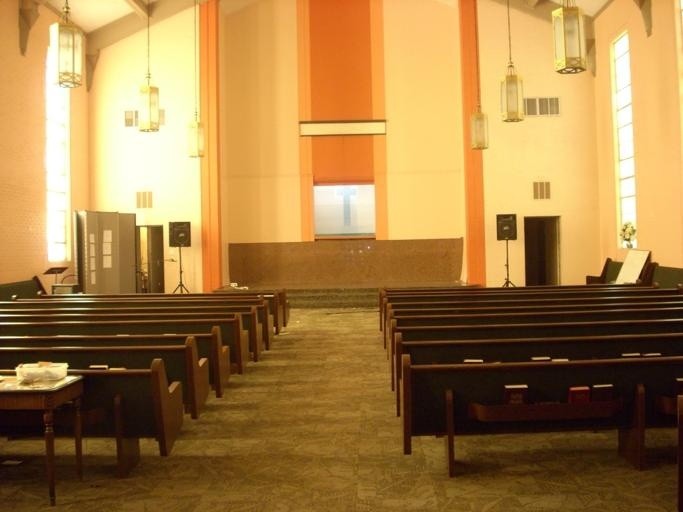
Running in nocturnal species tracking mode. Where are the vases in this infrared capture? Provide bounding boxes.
[626,242,633,248]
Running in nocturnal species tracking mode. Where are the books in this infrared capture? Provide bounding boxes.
[641,352,662,358]
[463,358,483,364]
[592,383,614,400]
[620,352,640,359]
[551,358,570,363]
[88,364,108,371]
[109,367,127,370]
[566,385,590,404]
[673,377,682,398]
[530,356,551,362]
[502,383,528,405]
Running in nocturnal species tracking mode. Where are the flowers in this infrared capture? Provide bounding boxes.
[619,222,637,242]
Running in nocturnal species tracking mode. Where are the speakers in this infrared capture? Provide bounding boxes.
[496,214,517,240]
[169,222,190,247]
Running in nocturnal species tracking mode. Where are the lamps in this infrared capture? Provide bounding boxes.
[49,1,207,159]
[468,0,587,149]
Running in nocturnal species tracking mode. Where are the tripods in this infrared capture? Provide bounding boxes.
[173,247,190,294]
[502,241,516,287]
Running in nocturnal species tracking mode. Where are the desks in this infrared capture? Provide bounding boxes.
[0,374,85,506]
